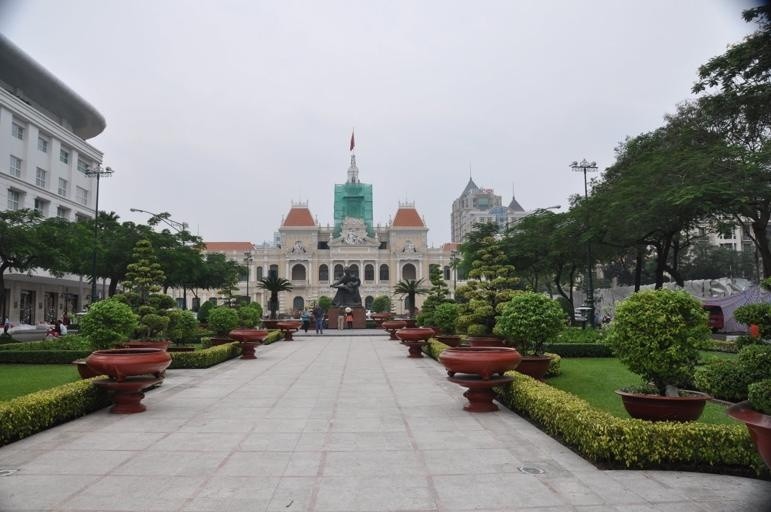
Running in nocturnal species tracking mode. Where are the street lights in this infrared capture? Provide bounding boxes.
[448,248,459,290]
[129,206,188,310]
[569,158,598,327]
[84,161,116,300]
[242,249,254,305]
[58,288,72,313]
[506,204,563,236]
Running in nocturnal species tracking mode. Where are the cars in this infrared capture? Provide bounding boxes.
[263,312,290,320]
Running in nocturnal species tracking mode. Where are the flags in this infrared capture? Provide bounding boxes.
[350,131,354,151]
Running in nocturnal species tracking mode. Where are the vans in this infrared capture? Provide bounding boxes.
[701,304,724,331]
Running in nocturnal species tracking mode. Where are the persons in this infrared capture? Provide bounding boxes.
[347,228,358,244]
[52,318,61,335]
[330,267,362,308]
[58,320,67,336]
[337,305,345,330]
[62,311,68,325]
[312,304,324,334]
[345,307,354,328]
[4,316,12,338]
[301,306,311,332]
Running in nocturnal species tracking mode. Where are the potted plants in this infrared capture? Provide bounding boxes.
[71,232,263,380]
[727,303,771,470]
[415,239,571,383]
[599,287,712,423]
